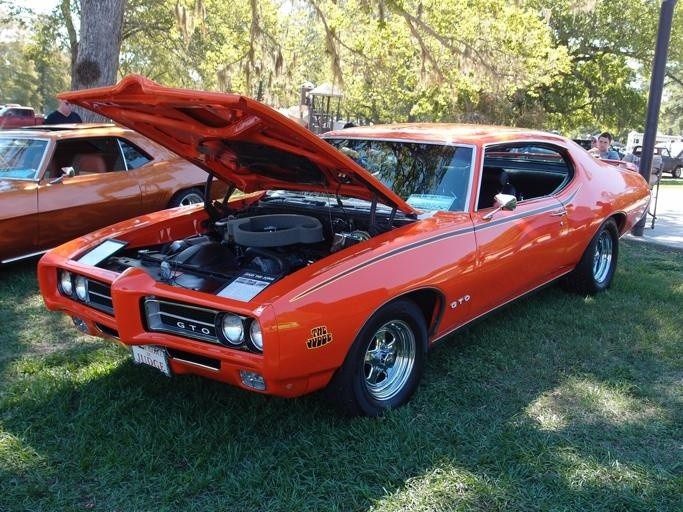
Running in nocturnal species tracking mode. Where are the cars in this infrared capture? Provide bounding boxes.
[576,129,682,179]
[33,73,653,415]
[0,123,230,271]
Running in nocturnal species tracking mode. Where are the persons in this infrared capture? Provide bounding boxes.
[585,132,620,162]
[43,98,82,122]
[590,134,600,149]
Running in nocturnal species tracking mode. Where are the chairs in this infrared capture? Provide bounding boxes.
[70,151,107,176]
[32,149,56,177]
[478,167,517,209]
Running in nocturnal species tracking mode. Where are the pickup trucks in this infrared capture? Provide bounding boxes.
[0,105,45,130]
